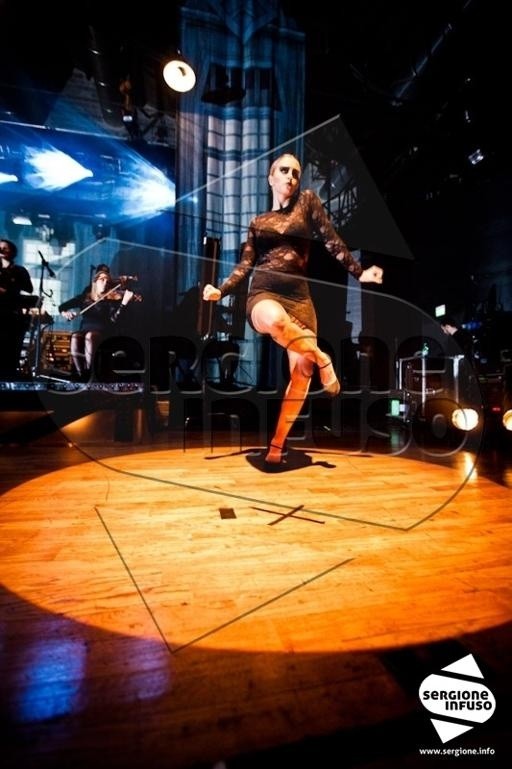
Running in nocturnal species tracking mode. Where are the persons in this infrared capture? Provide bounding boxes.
[176,285,240,387]
[439,317,482,405]
[201,150,387,474]
[82,264,129,298]
[57,270,134,383]
[1,237,33,381]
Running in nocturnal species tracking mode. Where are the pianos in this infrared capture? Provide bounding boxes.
[0,309,54,324]
[395,354,480,371]
[0,293,42,308]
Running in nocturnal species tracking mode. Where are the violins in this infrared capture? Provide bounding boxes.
[99,291,142,301]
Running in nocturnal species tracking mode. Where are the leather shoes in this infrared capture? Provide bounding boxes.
[317,352,342,400]
[263,441,283,472]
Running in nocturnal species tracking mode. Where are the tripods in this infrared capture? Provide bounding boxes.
[15,251,72,383]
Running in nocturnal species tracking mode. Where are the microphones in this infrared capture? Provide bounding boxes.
[45,265,56,279]
